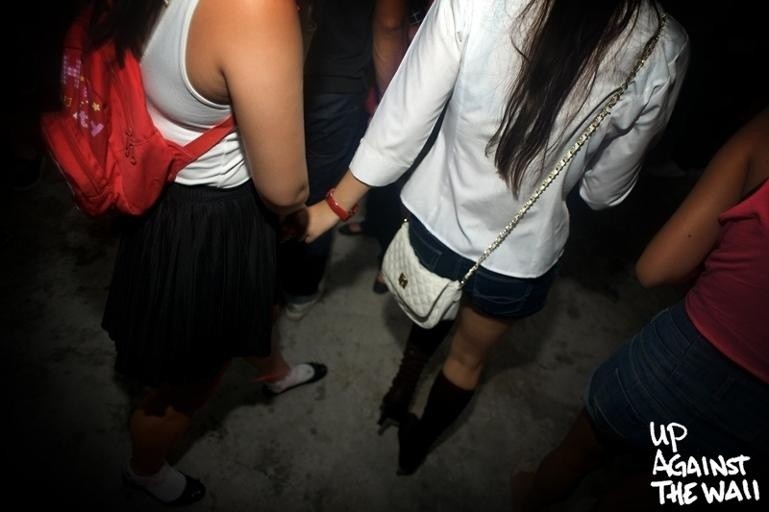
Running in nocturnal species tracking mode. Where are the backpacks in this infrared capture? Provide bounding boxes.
[38,0,238,219]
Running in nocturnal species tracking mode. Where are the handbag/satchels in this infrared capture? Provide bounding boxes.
[380,222,463,330]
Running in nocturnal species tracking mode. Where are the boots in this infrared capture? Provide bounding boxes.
[377,320,475,475]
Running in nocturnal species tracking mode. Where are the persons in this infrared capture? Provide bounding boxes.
[0,0,769,512]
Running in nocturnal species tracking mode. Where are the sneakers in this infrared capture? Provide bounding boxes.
[285,276,326,321]
[372,274,386,295]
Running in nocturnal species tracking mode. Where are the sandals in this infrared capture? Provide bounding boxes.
[338,222,370,235]
[119,471,204,506]
[262,363,328,396]
